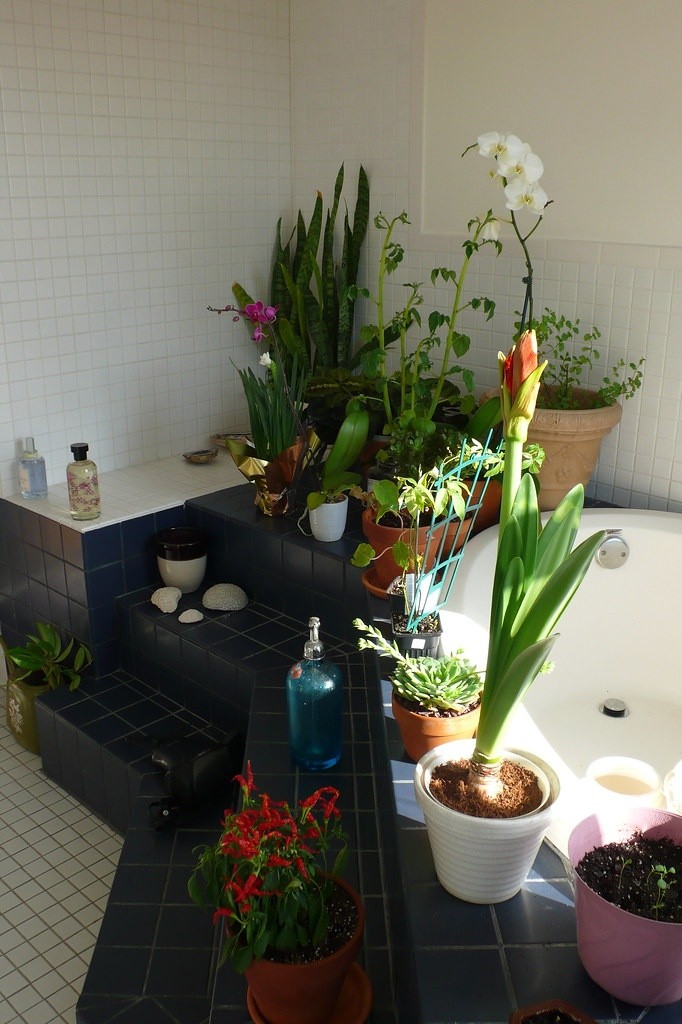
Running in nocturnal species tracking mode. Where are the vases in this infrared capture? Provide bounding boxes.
[305,493,356,542]
[414,739,562,904]
[156,526,210,596]
[479,385,624,511]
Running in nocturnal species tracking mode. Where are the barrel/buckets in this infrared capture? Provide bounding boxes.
[157,528,206,594]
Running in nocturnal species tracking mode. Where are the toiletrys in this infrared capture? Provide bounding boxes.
[16,431,49,503]
[285,614,347,774]
[61,439,103,523]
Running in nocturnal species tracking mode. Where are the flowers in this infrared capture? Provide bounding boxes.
[205,300,368,511]
[468,325,622,799]
[463,130,649,410]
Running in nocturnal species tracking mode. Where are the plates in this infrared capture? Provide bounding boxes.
[212,432,253,448]
[183,449,217,463]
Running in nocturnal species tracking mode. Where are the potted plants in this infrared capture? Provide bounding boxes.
[188,760,372,1024]
[568,805,682,1007]
[0,620,96,755]
[230,160,576,771]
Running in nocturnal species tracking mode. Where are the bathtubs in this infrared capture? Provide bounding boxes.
[434,507,681,855]
